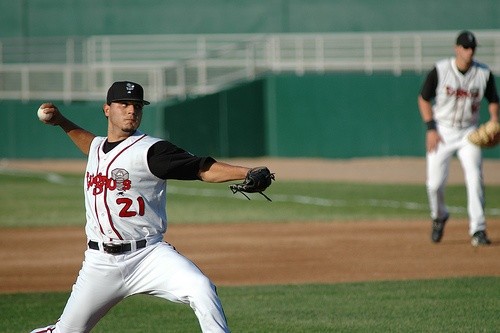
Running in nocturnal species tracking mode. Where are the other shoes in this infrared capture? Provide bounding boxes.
[431,213,449,242]
[471,232,491,246]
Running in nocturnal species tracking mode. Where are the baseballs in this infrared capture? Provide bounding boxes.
[37,105,53,122]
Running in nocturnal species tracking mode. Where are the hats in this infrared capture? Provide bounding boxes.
[107,82,150,105]
[457,31,476,47]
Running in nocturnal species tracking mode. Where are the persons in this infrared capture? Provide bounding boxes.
[417,32,500,245]
[30,81,272,333]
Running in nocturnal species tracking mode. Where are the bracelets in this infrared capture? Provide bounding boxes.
[426,120,436,130]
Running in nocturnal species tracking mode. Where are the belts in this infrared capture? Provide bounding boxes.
[88,240,146,255]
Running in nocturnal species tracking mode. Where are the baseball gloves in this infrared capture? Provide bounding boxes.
[468,120,500,149]
[228,168,272,194]
